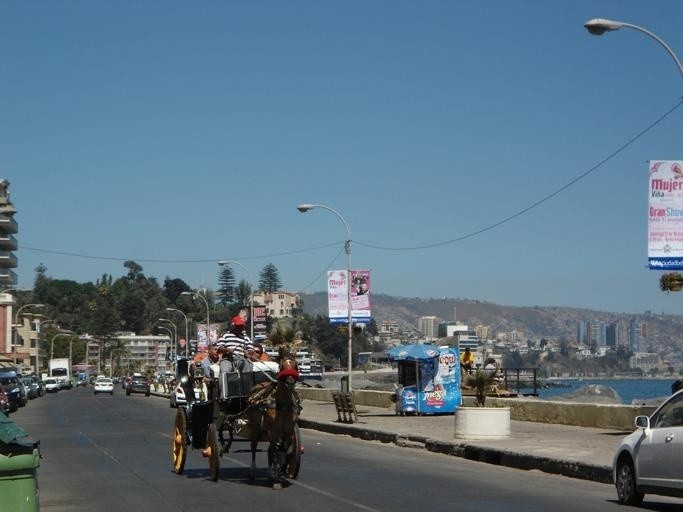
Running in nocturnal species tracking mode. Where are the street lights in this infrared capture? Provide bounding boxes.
[217,260,254,342]
[0,288,122,377]
[156,287,210,358]
[296,204,353,394]
[585,18,683,80]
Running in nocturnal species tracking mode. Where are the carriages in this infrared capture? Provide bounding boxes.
[171,347,304,489]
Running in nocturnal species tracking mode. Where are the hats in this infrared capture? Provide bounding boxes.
[277,357,300,381]
[230,316,245,326]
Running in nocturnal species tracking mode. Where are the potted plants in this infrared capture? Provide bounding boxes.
[452,368,512,441]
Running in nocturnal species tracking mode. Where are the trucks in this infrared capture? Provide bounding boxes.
[48,357,72,389]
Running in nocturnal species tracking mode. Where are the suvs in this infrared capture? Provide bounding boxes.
[125,376,150,397]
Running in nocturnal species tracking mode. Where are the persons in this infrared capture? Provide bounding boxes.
[200,342,220,384]
[250,343,262,362]
[459,346,474,370]
[483,357,504,385]
[215,315,255,406]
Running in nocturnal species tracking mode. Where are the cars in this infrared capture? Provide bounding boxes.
[0,367,44,415]
[43,377,59,392]
[76,369,141,388]
[611,387,683,506]
[169,380,202,408]
[93,378,113,395]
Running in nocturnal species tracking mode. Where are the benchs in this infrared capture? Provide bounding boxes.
[332,393,370,425]
[207,361,280,397]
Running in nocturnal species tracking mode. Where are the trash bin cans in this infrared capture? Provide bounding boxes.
[0,449,39,512]
